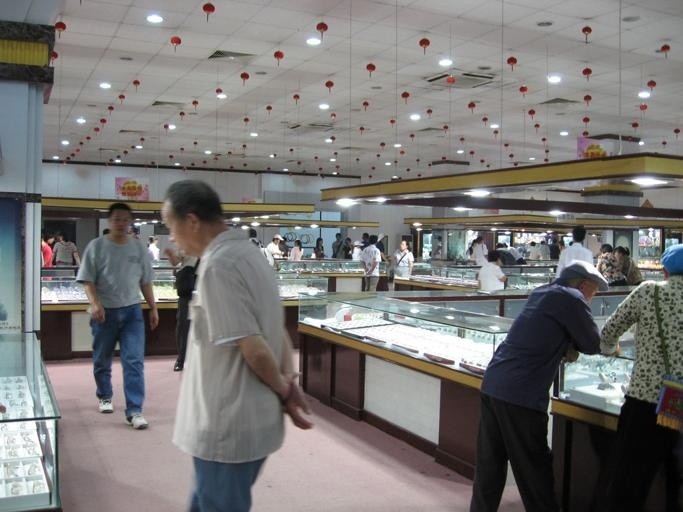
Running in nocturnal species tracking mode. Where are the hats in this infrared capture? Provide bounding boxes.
[377,234,385,242]
[274,234,284,241]
[560,261,609,291]
[353,241,365,246]
[660,244,683,273]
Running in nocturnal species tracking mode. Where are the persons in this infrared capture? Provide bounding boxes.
[166,246,199,373]
[392,241,414,275]
[75,204,160,430]
[468,256,610,511]
[147,236,159,261]
[598,245,644,287]
[160,179,314,511]
[290,240,304,260]
[314,238,327,259]
[477,251,507,289]
[495,239,573,265]
[249,229,288,266]
[466,236,488,264]
[41,229,80,281]
[555,225,594,280]
[332,232,387,291]
[127,228,140,240]
[103,229,110,235]
[597,244,682,512]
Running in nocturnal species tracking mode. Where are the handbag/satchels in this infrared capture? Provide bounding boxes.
[176,266,198,299]
[657,375,683,432]
[389,270,394,282]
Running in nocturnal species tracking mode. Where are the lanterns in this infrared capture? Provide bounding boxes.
[662,128,680,148]
[65,3,216,168]
[630,42,669,144]
[216,21,550,179]
[50,20,67,66]
[581,24,592,137]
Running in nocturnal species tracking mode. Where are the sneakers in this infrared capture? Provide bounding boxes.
[98,398,114,413]
[128,413,147,429]
